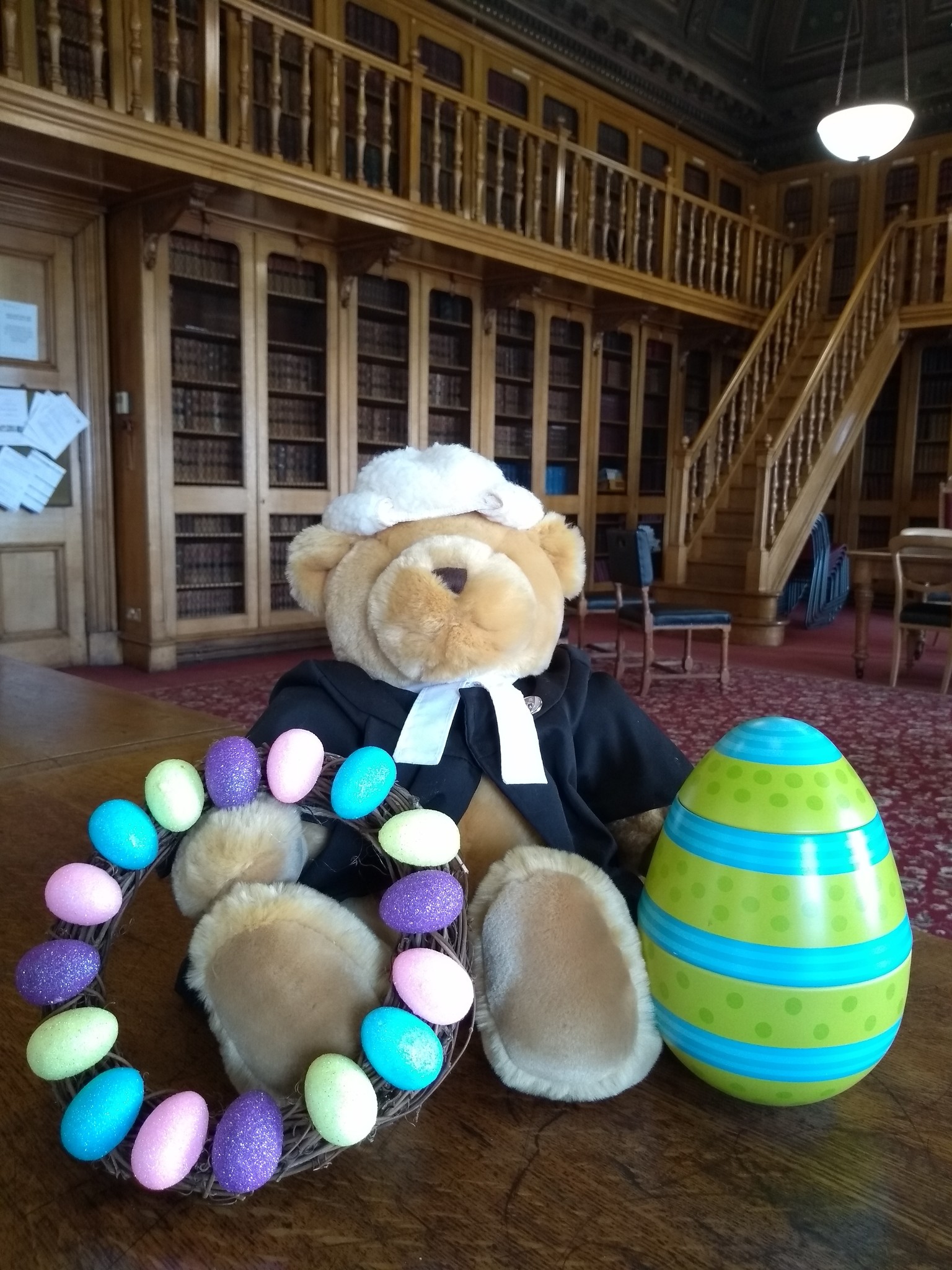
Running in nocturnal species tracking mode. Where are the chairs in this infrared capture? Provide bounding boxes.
[778,512,855,632]
[564,586,656,675]
[888,525,952,698]
[606,528,733,702]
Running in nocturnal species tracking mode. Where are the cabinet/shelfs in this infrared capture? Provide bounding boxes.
[0,0,767,661]
[776,135,952,605]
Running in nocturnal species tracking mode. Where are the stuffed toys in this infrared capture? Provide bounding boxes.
[171,439,699,1109]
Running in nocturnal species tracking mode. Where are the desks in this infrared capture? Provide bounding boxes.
[846,544,951,680]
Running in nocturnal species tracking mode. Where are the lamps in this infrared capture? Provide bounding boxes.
[817,0,915,162]
